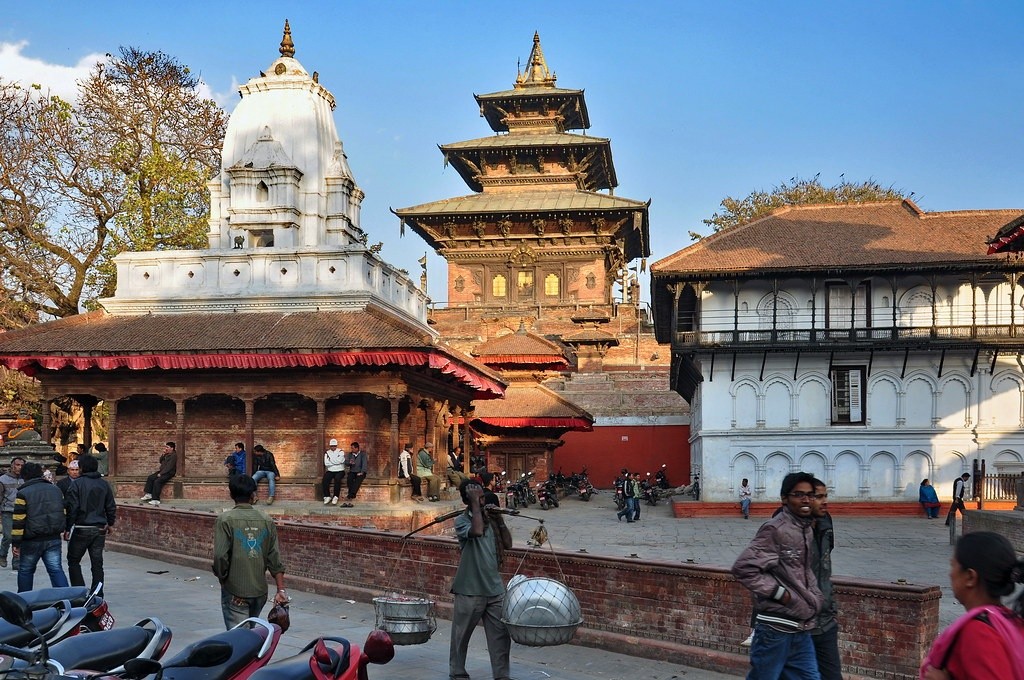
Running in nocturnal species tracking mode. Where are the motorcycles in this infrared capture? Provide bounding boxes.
[612,462,668,510]
[506,464,593,510]
[0,582,394,680]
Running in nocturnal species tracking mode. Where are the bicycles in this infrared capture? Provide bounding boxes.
[689,471,701,500]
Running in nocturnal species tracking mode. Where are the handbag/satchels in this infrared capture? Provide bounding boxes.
[268,601,290,634]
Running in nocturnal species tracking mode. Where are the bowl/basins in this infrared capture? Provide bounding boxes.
[373,597,436,620]
[379,620,434,646]
[502,576,581,625]
[501,617,585,647]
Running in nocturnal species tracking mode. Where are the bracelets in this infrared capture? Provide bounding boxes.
[277,585,285,593]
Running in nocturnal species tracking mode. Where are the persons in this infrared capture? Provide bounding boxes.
[740,478,843,680]
[344,442,368,501]
[617,472,641,523]
[731,471,825,680]
[945,473,970,525]
[0,442,116,600]
[322,439,346,505]
[481,472,501,508]
[739,478,752,519]
[397,443,424,502]
[141,442,177,505]
[210,474,291,630]
[921,530,1024,680]
[250,444,280,505]
[447,447,468,488]
[919,479,941,519]
[448,480,513,680]
[416,442,441,502]
[223,442,246,475]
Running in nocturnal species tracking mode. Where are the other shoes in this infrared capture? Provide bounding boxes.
[266,497,274,505]
[617,512,622,521]
[12,562,20,570]
[141,493,152,500]
[928,516,932,519]
[410,494,424,503]
[633,518,640,520]
[0,556,7,567]
[340,502,354,508]
[148,500,160,505]
[332,496,339,505]
[324,497,332,505]
[627,520,636,523]
[426,495,438,502]
[253,496,259,504]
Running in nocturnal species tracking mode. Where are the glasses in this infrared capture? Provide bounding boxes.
[787,490,816,500]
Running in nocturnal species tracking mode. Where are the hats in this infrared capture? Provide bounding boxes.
[329,439,337,446]
[405,442,413,448]
[68,460,79,468]
[424,442,434,448]
